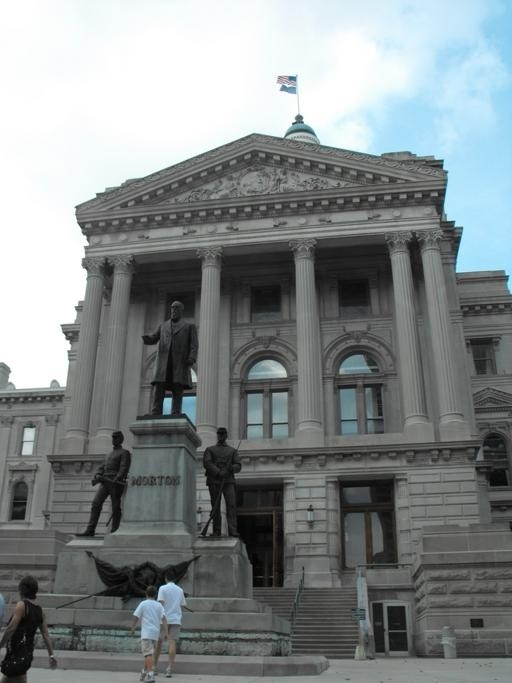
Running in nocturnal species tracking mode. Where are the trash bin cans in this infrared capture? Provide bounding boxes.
[442,626,457,659]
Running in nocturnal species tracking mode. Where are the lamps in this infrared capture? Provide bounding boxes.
[196,506,203,527]
[305,504,314,528]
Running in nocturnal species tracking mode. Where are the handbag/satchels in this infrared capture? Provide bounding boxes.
[0,637,34,677]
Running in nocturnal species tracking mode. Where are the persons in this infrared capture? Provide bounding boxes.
[202,427,243,538]
[141,300,199,416]
[130,570,188,683]
[75,430,132,538]
[0,575,58,683]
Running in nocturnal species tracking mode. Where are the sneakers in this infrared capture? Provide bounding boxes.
[166,669,172,676]
[139,667,157,682]
